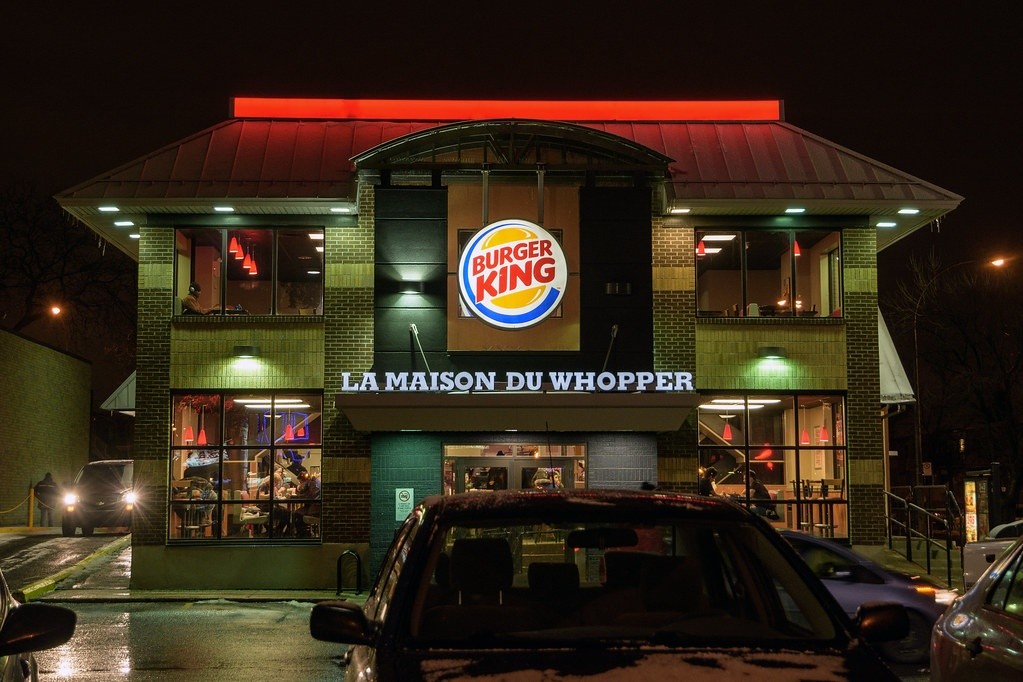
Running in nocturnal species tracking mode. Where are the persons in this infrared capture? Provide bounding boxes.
[535,469,566,490]
[293,471,321,538]
[182,282,209,315]
[34,472,59,528]
[742,470,774,516]
[287,455,308,477]
[254,471,291,538]
[699,464,726,500]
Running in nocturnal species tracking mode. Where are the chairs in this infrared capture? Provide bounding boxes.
[302,515,321,538]
[789,479,846,538]
[232,490,269,537]
[298,308,316,315]
[422,538,545,637]
[769,490,787,529]
[597,548,704,620]
[724,303,740,317]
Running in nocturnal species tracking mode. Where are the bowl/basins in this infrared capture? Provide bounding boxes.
[730,494,739,497]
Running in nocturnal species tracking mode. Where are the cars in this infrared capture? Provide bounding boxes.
[779,529,1023,682]
[0,568,77,682]
[311,491,902,682]
[62,460,135,537]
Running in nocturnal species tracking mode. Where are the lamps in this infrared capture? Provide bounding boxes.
[186,402,194,442]
[801,405,811,445]
[756,346,787,359]
[697,240,706,256]
[284,408,295,441]
[723,410,732,440]
[606,282,631,295]
[794,240,801,256]
[198,404,208,445]
[820,401,828,443]
[233,345,261,357]
[229,229,257,275]
[297,427,305,437]
[397,280,425,294]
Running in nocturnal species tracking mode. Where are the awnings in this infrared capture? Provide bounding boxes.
[100,369,136,417]
[877,303,917,407]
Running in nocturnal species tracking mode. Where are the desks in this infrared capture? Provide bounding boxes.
[759,310,817,317]
[210,309,248,316]
[696,309,723,317]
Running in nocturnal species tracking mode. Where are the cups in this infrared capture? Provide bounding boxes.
[722,491,726,496]
[287,487,296,496]
